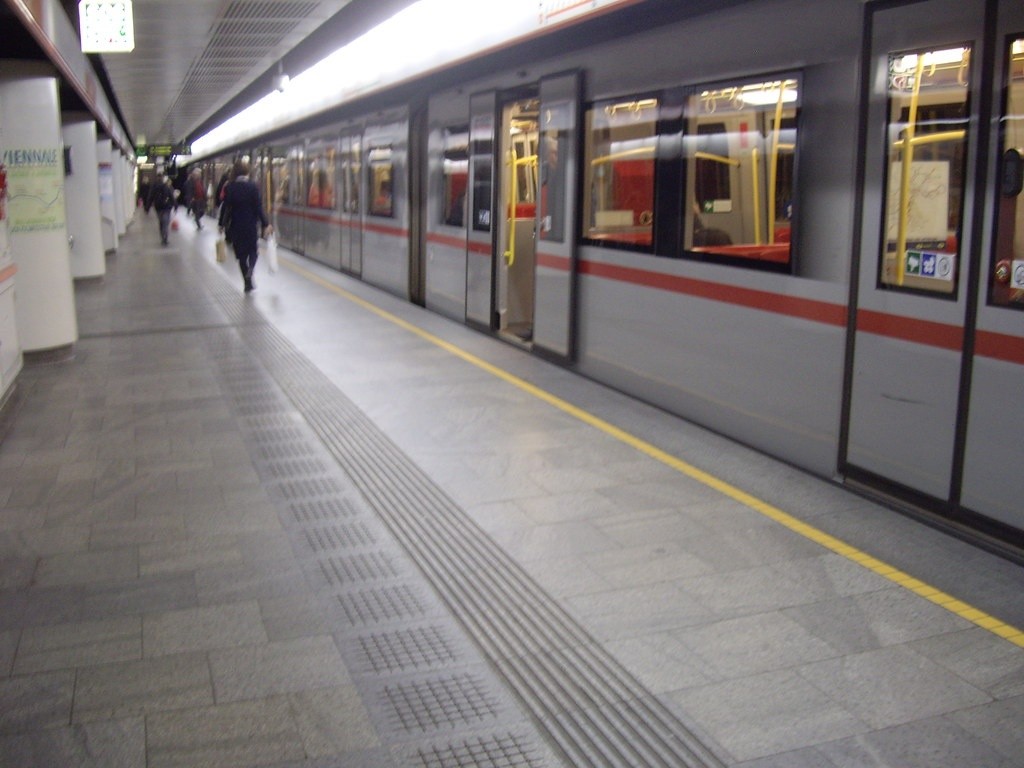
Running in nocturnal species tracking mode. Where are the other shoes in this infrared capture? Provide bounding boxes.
[244,278,253,291]
[162,236,168,246]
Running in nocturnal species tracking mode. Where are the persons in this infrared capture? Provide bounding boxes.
[218,163,273,293]
[215,167,238,260]
[148,173,178,247]
[189,168,209,230]
[276,165,332,255]
[140,175,153,213]
[183,175,193,216]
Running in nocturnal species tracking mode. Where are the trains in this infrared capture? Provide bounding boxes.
[176,2,1024,565]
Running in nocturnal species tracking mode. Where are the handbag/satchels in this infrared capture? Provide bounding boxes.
[171,213,180,230]
[267,231,278,272]
[216,232,226,261]
